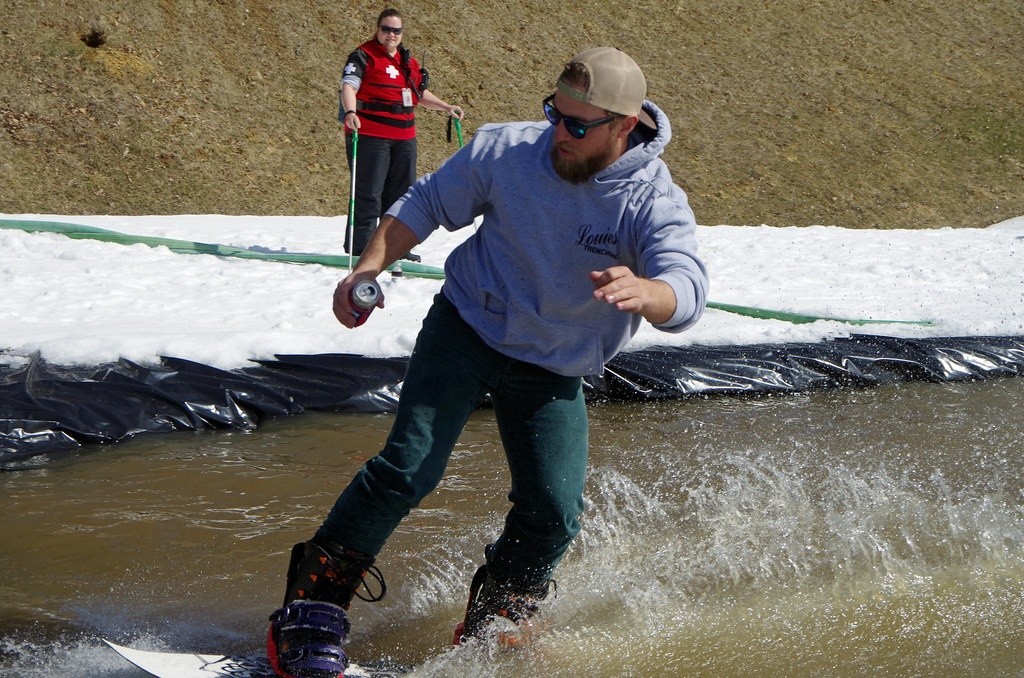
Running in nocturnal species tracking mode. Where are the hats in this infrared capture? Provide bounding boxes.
[557,47,657,130]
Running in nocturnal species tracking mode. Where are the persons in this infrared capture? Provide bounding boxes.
[340,9,464,263]
[268,48,708,678]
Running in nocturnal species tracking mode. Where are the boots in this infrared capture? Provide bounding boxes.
[272,542,386,678]
[464,544,559,651]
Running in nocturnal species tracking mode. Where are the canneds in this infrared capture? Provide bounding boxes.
[349,281,381,327]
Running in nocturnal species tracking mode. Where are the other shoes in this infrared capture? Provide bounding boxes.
[400,253,421,262]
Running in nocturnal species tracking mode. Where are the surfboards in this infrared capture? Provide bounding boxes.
[101,636,540,678]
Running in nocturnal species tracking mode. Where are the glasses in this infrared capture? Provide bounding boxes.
[378,25,404,35]
[543,92,615,139]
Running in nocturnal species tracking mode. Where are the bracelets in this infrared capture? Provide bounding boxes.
[344,110,357,118]
